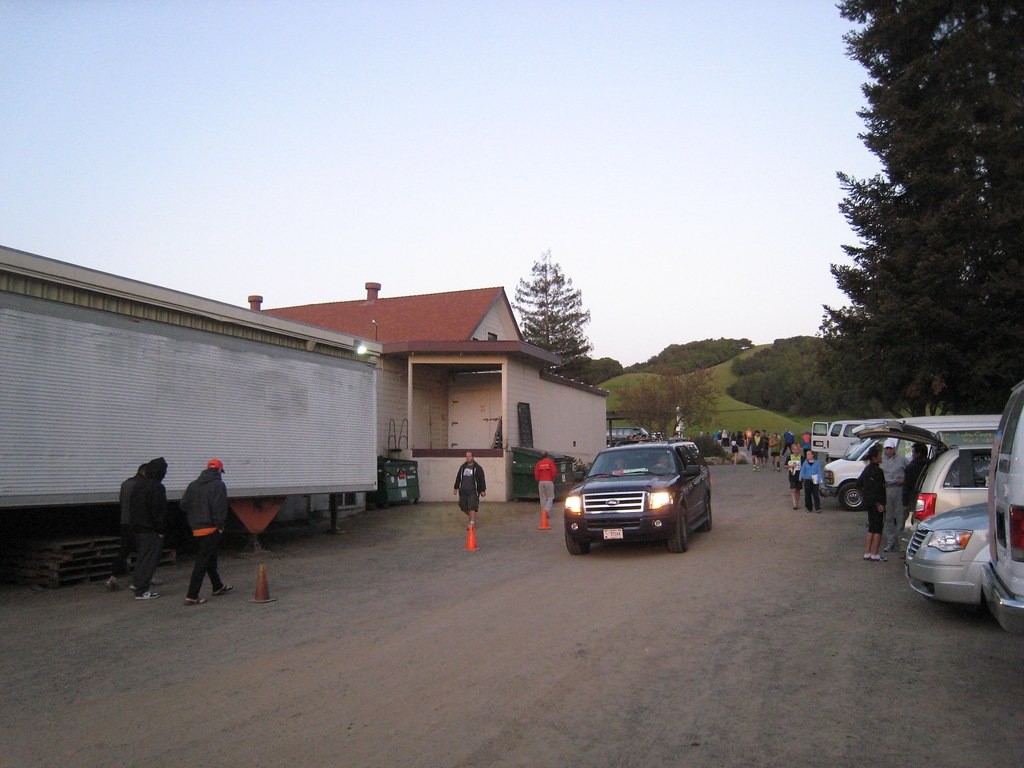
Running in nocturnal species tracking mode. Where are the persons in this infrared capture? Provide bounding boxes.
[130,457,168,600]
[180,459,234,606]
[732,443,738,466]
[714,427,753,450]
[454,451,486,531]
[802,430,811,457]
[656,455,669,470]
[902,443,928,512]
[799,449,822,513]
[747,429,781,473]
[780,429,794,458]
[613,455,628,470]
[674,433,678,438]
[785,444,803,510]
[879,439,910,553]
[627,435,646,440]
[688,436,692,442]
[104,463,163,591]
[857,447,888,562]
[535,453,557,519]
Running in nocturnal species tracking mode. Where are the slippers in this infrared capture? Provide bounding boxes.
[186,597,208,606]
[213,584,234,596]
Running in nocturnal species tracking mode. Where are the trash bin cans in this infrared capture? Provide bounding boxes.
[365,456,420,509]
[511,446,575,499]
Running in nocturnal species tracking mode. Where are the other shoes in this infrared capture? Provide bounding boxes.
[863,555,871,559]
[793,504,800,509]
[105,579,119,590]
[817,508,823,512]
[870,555,888,561]
[807,507,813,512]
[750,462,781,472]
[148,578,162,585]
[467,521,476,530]
[884,543,896,551]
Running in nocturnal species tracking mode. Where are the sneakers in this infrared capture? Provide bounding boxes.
[134,590,160,600]
[129,584,136,589]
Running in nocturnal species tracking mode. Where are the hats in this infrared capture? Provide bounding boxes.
[207,458,225,473]
[882,440,896,449]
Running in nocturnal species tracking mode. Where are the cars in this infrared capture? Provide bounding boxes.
[902,500,992,626]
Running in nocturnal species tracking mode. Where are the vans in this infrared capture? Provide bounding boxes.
[810,413,1007,541]
[979,379,1024,638]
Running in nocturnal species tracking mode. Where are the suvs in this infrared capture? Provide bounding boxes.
[562,436,714,555]
[605,427,648,447]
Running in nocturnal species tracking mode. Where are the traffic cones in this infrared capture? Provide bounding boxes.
[460,521,481,552]
[249,563,276,604]
[537,507,553,530]
